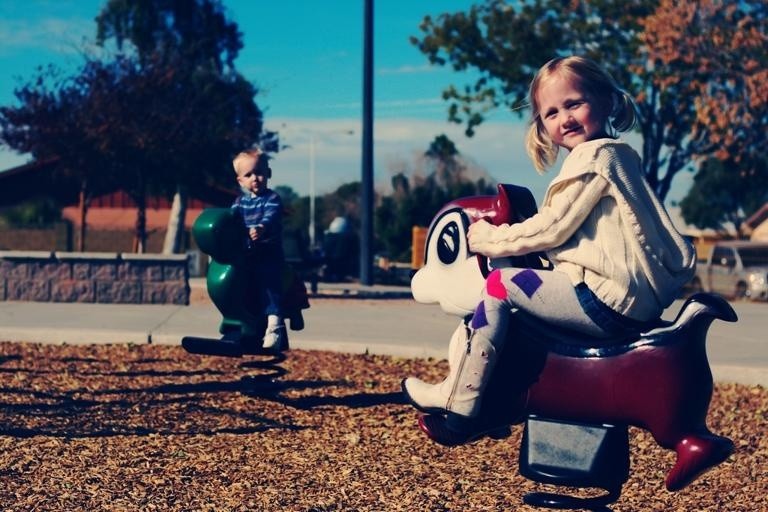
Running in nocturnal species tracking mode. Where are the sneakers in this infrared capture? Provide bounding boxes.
[261,323,285,349]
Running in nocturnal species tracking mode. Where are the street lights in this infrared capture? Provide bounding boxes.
[309,129,354,246]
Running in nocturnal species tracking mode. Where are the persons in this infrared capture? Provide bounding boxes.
[225,141,288,352]
[398,50,700,423]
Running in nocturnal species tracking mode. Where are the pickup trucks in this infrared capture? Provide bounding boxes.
[695,240,768,303]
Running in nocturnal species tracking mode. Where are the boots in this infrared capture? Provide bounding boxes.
[400,325,496,417]
